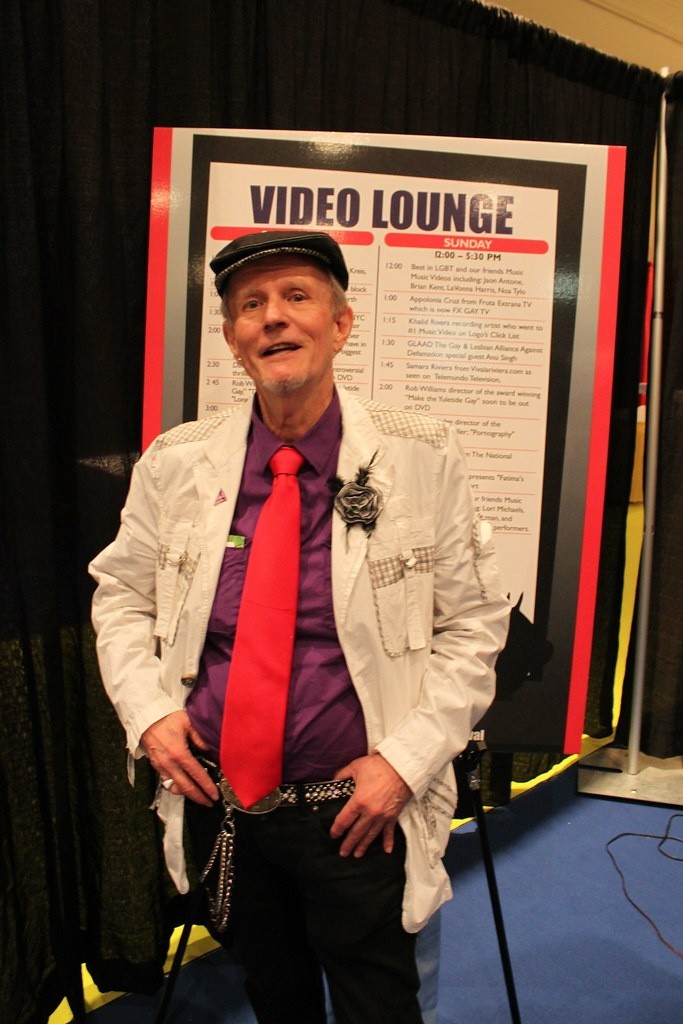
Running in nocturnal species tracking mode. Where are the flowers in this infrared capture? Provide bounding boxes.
[324,446,386,538]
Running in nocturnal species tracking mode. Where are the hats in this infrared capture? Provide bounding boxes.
[210,231,348,301]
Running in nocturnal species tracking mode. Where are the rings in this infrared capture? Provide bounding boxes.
[162,778,173,790]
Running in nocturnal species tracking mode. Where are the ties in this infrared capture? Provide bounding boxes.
[221,447,299,810]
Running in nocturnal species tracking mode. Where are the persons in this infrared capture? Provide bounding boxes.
[88,232,511,1024]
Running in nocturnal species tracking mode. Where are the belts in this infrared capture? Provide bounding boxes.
[197,759,357,814]
